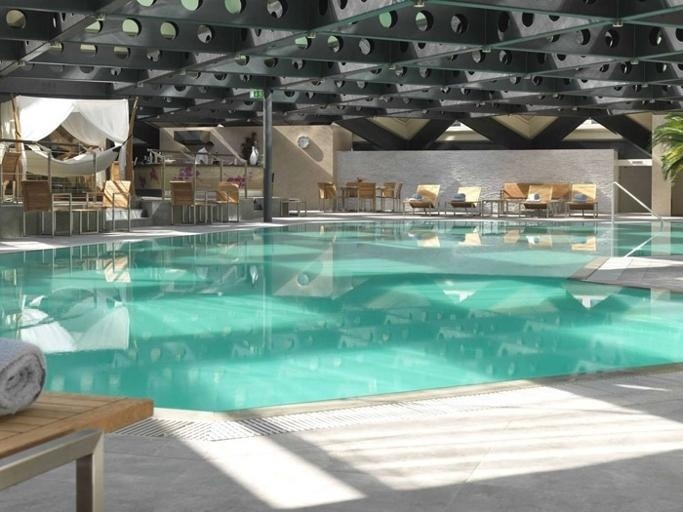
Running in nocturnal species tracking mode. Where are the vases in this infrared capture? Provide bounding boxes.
[249,146,258,165]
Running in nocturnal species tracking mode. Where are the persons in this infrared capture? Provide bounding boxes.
[194,141,214,165]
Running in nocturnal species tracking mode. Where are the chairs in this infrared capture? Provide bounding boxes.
[215,181,240,223]
[94,242,131,283]
[23,249,56,296]
[84,180,132,232]
[565,183,597,218]
[410,184,441,216]
[450,186,482,217]
[519,185,554,217]
[408,221,596,252]
[0,387,152,512]
[21,180,73,236]
[0,151,22,206]
[169,180,198,226]
[317,181,402,213]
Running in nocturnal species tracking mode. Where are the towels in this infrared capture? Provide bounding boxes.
[0,337,46,416]
